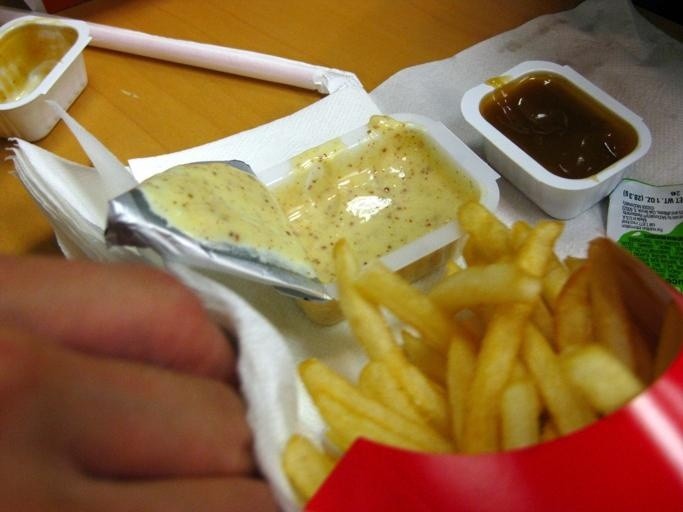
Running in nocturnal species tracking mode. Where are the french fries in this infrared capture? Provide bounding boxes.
[281,202,679,507]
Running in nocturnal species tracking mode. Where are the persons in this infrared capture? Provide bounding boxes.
[0,257,285,512]
